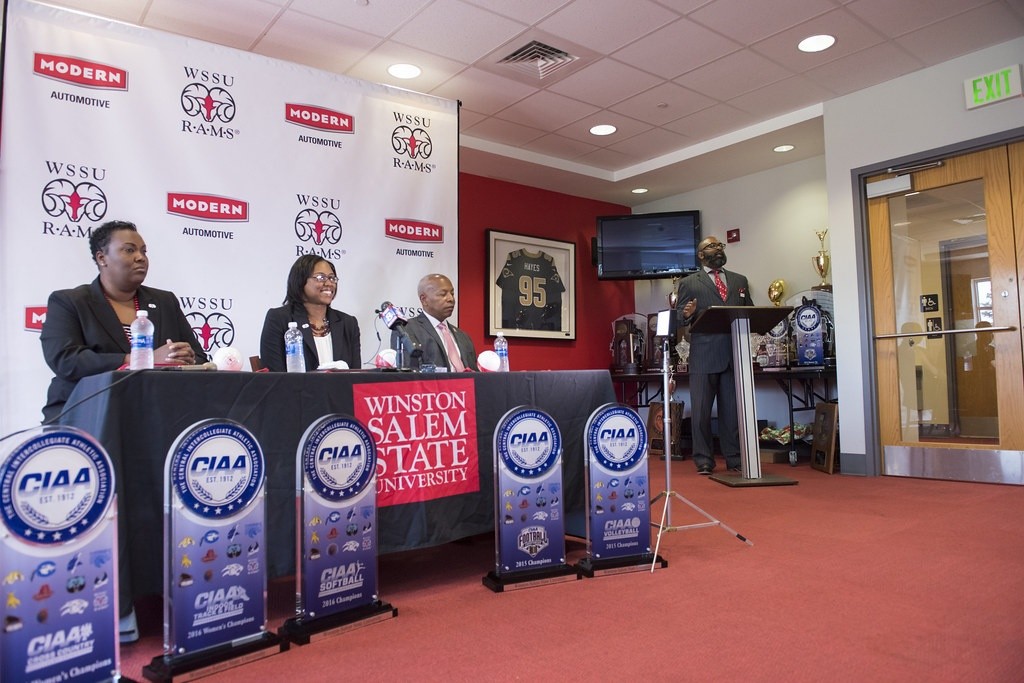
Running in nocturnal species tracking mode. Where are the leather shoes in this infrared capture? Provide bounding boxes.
[728,465,742,472]
[697,462,713,474]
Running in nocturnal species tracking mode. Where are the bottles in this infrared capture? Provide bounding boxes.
[130,310,154,370]
[494,332,510,373]
[283,321,306,373]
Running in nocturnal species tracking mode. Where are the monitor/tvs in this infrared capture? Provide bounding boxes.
[596,209,701,281]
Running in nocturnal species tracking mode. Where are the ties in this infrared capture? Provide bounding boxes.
[438,323,465,373]
[713,270,728,302]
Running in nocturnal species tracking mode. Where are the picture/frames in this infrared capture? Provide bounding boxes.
[483,227,577,345]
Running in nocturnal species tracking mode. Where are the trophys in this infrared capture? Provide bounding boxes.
[810,228,830,287]
[676,334,690,374]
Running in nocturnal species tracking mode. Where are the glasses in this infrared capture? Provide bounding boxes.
[306,276,339,284]
[700,243,726,252]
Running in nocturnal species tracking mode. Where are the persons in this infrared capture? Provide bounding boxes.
[897,323,946,407]
[389,273,478,373]
[959,322,994,370]
[39,220,212,431]
[674,236,757,475]
[259,254,360,371]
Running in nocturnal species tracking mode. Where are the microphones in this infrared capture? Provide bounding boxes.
[380,301,424,358]
[375,310,382,313]
[161,363,218,371]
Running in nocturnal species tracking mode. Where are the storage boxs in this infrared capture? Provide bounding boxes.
[760,449,788,463]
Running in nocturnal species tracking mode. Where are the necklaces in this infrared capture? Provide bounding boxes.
[310,320,330,338]
[103,293,140,346]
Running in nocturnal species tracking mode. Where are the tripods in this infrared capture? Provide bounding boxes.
[650,340,748,575]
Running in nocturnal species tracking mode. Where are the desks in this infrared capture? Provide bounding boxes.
[611,360,836,467]
[61,361,619,620]
